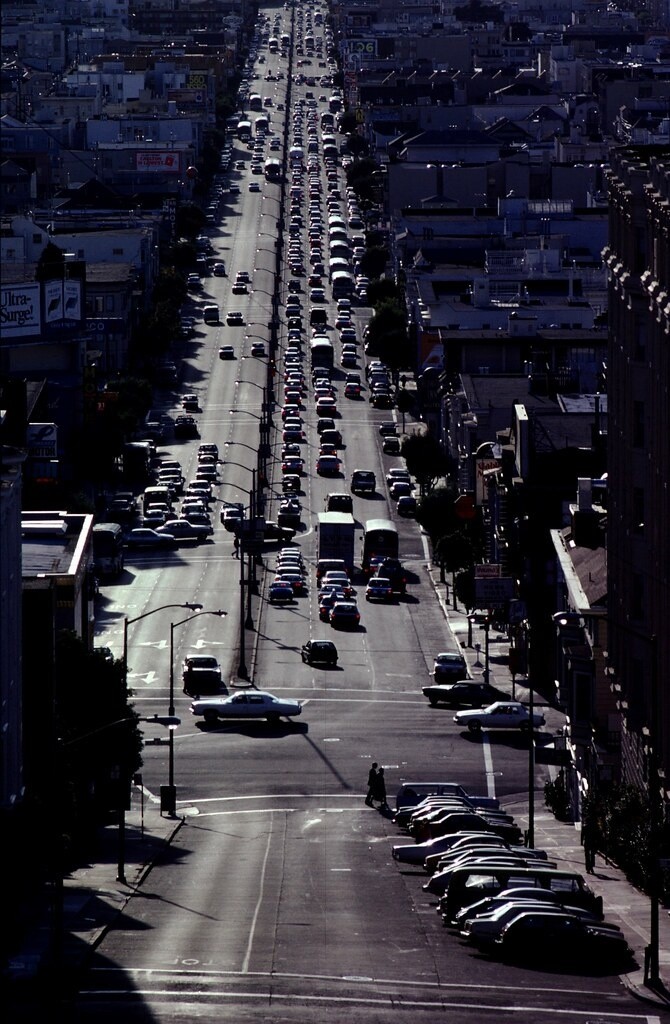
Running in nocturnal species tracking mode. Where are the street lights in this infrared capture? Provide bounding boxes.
[116,603,203,884]
[197,0,327,690]
[168,611,228,817]
[51,715,182,921]
[553,609,664,991]
[467,614,536,851]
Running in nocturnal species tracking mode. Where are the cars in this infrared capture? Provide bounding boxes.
[389,781,635,973]
[182,639,548,732]
[96,0,421,629]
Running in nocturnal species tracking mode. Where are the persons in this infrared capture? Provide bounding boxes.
[554,770,564,792]
[231,535,240,559]
[365,763,378,808]
[376,768,391,811]
[581,817,600,874]
